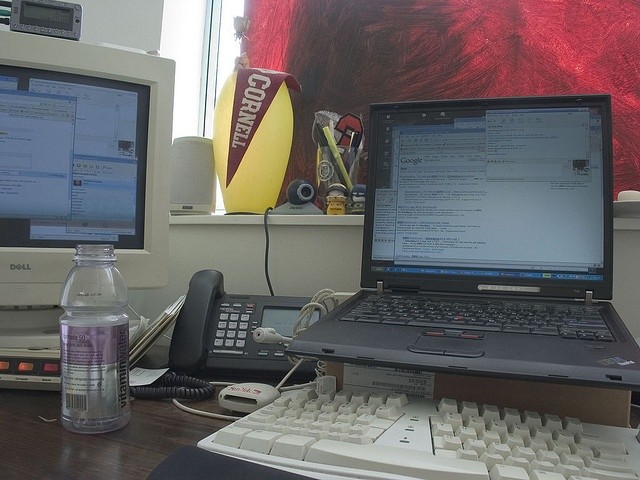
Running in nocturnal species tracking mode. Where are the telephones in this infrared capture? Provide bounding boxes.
[129,268,325,400]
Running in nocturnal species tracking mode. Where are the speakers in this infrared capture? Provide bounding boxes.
[170,136,215,215]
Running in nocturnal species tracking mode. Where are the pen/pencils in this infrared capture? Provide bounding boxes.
[323,126,356,195]
[314,121,349,193]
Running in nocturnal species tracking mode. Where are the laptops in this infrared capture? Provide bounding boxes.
[283,94,640,387]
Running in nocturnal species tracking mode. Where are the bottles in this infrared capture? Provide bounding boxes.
[58,244,130,434]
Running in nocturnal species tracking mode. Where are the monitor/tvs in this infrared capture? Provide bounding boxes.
[1,29,176,307]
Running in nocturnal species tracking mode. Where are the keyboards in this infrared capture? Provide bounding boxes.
[196,381,640,480]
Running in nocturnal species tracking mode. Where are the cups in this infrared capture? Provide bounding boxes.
[312,146,359,212]
